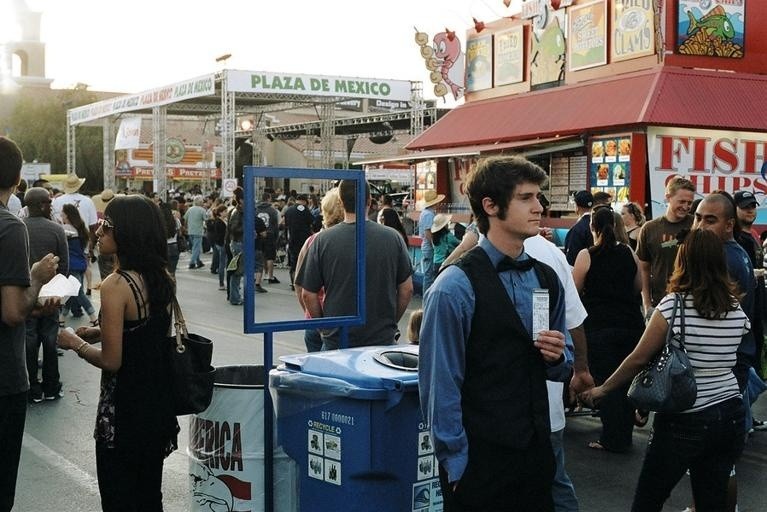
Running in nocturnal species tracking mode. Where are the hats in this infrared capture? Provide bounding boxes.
[429,213,453,233]
[572,189,593,209]
[60,173,87,194]
[416,187,445,210]
[92,186,125,212]
[734,190,761,209]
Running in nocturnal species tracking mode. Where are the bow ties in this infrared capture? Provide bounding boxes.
[496,255,537,273]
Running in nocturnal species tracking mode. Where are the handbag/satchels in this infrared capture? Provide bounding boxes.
[226,252,246,277]
[626,291,697,413]
[153,266,219,418]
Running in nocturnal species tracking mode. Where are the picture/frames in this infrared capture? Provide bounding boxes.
[567,1,608,73]
[493,24,524,88]
[463,33,492,92]
[675,1,747,60]
[608,0,655,66]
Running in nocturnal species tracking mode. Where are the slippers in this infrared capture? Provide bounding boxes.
[586,435,634,453]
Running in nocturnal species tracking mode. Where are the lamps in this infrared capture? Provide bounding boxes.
[266,133,275,142]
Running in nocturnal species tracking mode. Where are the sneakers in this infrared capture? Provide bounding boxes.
[29,347,66,406]
[746,411,767,435]
[188,262,297,306]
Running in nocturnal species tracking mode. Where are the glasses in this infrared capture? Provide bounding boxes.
[31,197,52,206]
[92,215,119,234]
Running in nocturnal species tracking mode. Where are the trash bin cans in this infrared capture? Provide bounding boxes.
[271,343,442,512]
